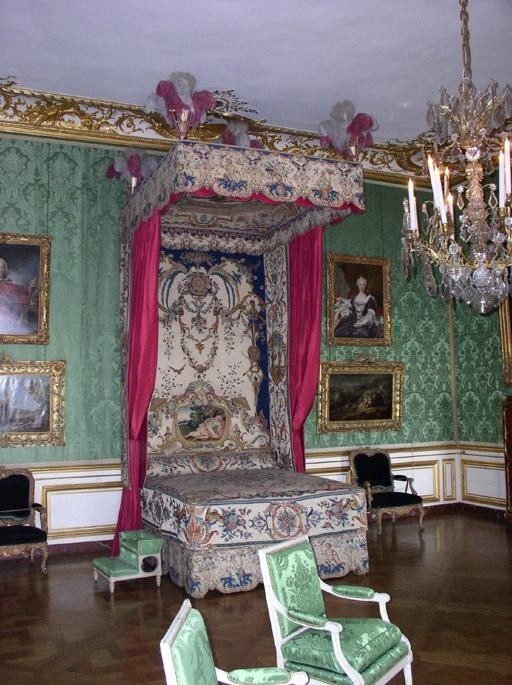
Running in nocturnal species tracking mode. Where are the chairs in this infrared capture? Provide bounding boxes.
[160,598,309,685]
[0,467,50,575]
[258,533,414,685]
[347,449,427,534]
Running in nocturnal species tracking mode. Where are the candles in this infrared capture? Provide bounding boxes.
[427,154,439,208]
[409,195,418,237]
[432,164,447,222]
[504,135,511,192]
[499,149,505,207]
[407,176,416,229]
[444,165,448,210]
[447,192,453,223]
[394,0,512,317]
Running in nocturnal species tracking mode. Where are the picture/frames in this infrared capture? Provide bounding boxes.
[323,252,395,348]
[317,359,405,435]
[0,358,68,450]
[0,231,52,347]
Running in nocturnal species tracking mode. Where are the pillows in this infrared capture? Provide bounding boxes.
[144,450,278,478]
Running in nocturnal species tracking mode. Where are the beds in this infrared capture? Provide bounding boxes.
[122,378,371,599]
